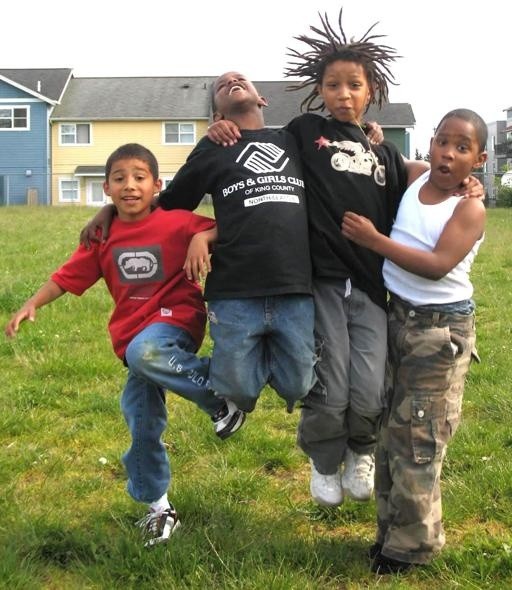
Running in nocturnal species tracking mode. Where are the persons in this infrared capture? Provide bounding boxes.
[341,111,486,572]
[204,4,486,508]
[4,145,248,547]
[78,70,384,415]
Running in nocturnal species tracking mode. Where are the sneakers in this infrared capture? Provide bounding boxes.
[211,400,245,439]
[369,540,415,576]
[342,441,375,502]
[310,456,345,507]
[141,502,180,549]
